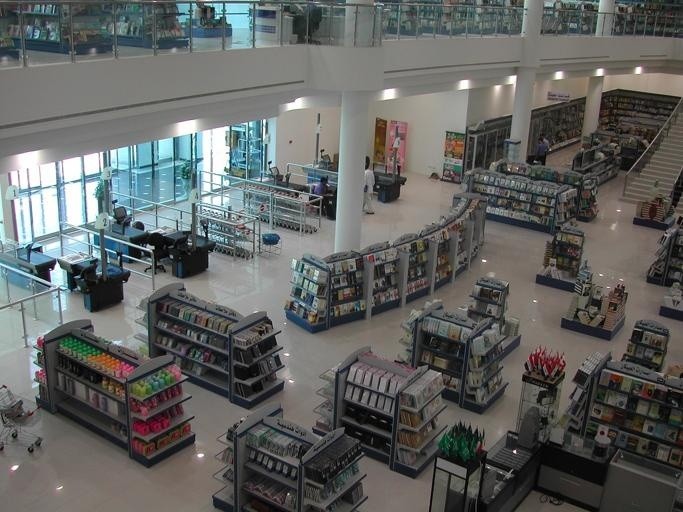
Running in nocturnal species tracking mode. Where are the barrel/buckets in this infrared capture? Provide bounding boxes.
[595,435,612,460]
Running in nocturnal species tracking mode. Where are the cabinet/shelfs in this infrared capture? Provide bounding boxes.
[134,282,287,409]
[311,345,448,480]
[31,318,198,471]
[227,159,408,219]
[645,218,682,289]
[463,160,620,234]
[621,318,672,374]
[427,351,681,512]
[1,0,184,42]
[396,298,509,414]
[468,275,522,357]
[284,189,488,334]
[210,403,369,512]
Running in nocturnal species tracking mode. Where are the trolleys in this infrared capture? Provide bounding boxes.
[0,384,43,452]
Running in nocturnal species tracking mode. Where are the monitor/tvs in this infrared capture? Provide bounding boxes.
[322,154,331,162]
[269,166,280,176]
[113,206,126,220]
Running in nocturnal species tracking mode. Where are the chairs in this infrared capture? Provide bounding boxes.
[144,233,167,275]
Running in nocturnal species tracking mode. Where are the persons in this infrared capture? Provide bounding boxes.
[540,134,549,152]
[553,0,563,18]
[536,138,547,166]
[315,175,333,218]
[364,163,376,213]
[284,0,316,43]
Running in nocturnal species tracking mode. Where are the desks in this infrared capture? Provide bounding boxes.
[92,221,147,264]
[57,251,130,314]
[162,225,216,279]
